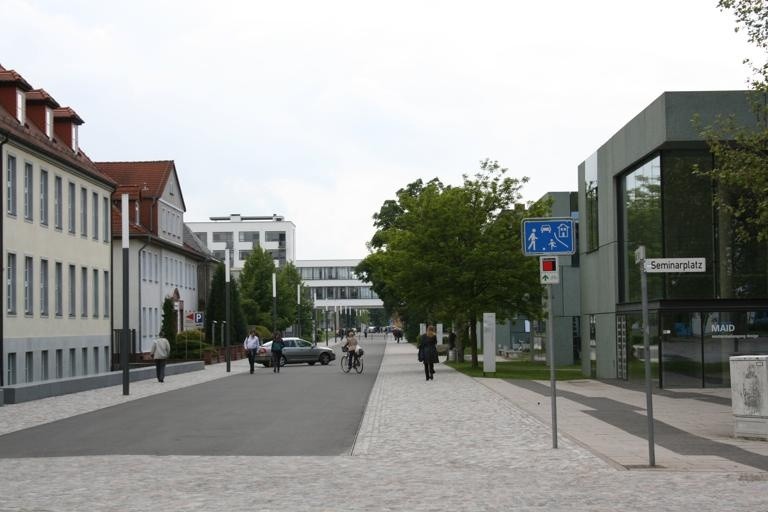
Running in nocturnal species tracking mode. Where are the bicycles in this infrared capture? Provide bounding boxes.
[340,345,364,374]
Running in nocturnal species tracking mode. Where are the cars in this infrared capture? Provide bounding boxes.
[367,326,377,333]
[254,337,336,367]
[323,327,358,334]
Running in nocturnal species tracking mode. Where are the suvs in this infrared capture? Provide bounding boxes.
[386,326,400,334]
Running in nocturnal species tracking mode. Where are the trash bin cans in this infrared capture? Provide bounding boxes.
[448,350,456,362]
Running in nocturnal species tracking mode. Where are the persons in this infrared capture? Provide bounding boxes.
[393,327,403,344]
[417,325,439,381]
[446,328,456,361]
[150,330,171,382]
[345,330,360,372]
[243,329,259,375]
[271,331,285,373]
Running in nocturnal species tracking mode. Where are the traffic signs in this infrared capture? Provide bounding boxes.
[645,257,706,273]
